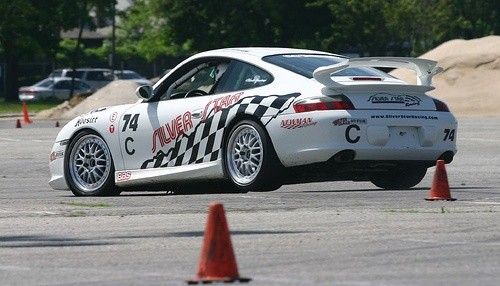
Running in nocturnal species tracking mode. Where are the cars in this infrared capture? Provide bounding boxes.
[18,76,97,104]
[115,69,152,86]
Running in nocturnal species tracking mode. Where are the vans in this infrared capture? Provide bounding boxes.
[49,68,121,90]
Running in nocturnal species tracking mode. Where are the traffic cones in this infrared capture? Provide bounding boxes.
[185,201,254,285]
[423,158,459,202]
[21,100,33,125]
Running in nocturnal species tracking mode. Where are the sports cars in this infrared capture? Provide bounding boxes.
[47,45,460,198]
